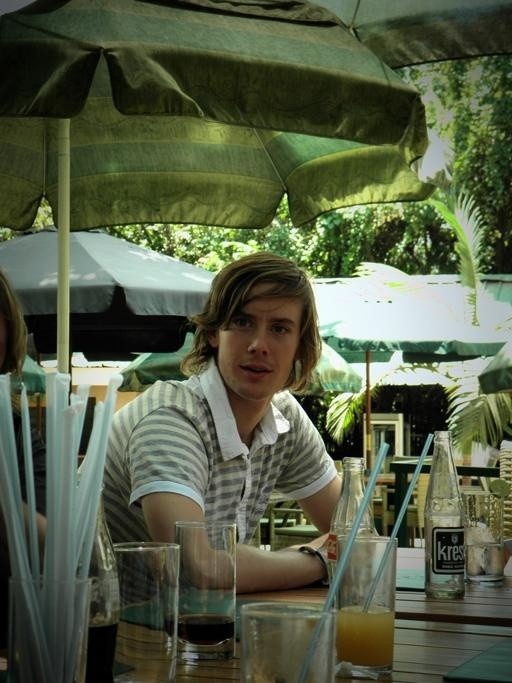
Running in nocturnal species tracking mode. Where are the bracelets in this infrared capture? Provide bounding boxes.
[300,543,332,583]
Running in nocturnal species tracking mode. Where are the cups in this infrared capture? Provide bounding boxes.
[173,517,238,661]
[462,487,505,586]
[331,532,401,677]
[9,574,92,682]
[239,601,337,682]
[109,539,182,681]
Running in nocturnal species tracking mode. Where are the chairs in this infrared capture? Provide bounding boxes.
[240,456,512,563]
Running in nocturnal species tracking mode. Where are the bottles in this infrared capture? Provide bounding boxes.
[328,454,375,607]
[73,479,120,682]
[422,428,467,600]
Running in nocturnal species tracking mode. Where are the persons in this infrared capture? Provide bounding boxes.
[0,271,47,552]
[105,252,378,590]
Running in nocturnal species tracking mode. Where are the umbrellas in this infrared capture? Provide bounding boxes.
[424,271,512,301]
[0,0,453,358]
[304,1,512,69]
[312,272,507,361]
[1,224,218,357]
[117,327,362,391]
